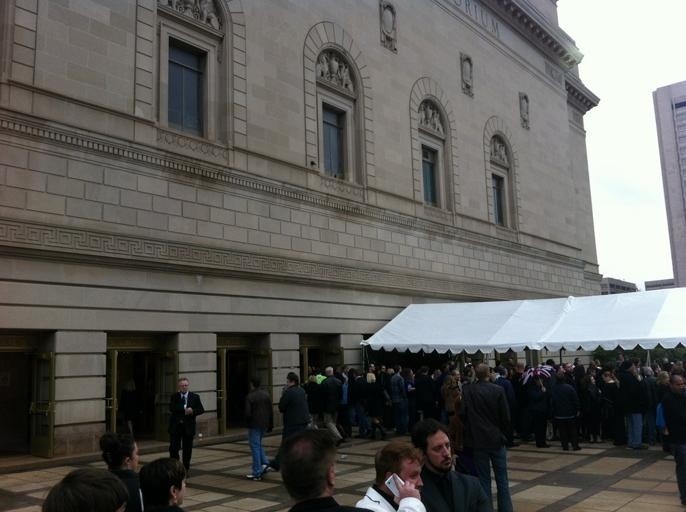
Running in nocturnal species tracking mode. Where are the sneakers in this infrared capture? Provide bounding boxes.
[627,442,650,449]
[246,462,271,482]
[562,445,583,451]
[535,442,552,448]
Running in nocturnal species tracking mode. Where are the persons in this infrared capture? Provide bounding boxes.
[465,361,514,510]
[357,441,427,512]
[138,458,187,512]
[430,371,511,477]
[168,378,205,473]
[515,356,685,455]
[100,432,142,511]
[245,373,273,479]
[41,468,130,512]
[412,418,492,511]
[280,427,373,511]
[278,358,514,446]
[660,375,685,505]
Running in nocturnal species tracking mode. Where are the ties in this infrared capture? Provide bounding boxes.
[181,394,186,405]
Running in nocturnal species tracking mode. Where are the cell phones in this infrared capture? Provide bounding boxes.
[385,472,405,497]
